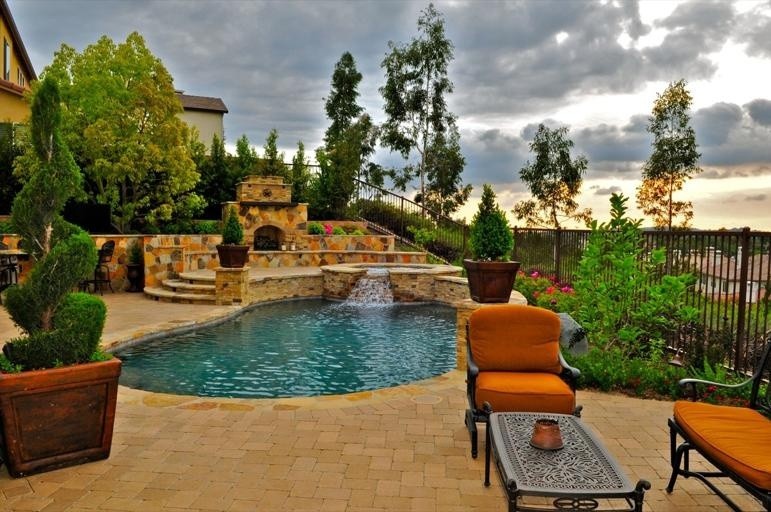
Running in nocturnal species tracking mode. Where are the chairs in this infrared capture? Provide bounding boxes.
[0,234,116,305]
[465,304,584,459]
[665,327,771,512]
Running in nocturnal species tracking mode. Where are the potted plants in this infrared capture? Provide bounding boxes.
[125,240,144,293]
[0,74,123,479]
[215,205,250,268]
[462,183,522,303]
